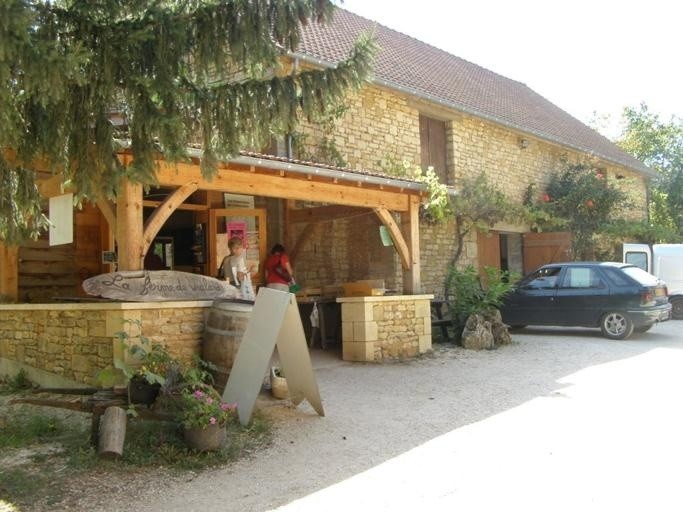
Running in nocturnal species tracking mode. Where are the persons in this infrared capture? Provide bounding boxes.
[218,236,258,288]
[263,241,296,293]
[144,244,164,270]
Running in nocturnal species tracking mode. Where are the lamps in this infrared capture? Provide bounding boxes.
[521,138,528,148]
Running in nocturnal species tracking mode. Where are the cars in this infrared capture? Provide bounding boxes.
[491,261,672,341]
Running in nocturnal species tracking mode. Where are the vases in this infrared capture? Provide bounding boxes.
[182,423,229,450]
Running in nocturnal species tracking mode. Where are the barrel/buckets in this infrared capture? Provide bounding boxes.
[202,300,255,397]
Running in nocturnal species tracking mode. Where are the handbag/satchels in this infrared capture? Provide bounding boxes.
[275,263,291,282]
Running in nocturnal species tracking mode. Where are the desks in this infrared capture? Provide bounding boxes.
[296,300,340,350]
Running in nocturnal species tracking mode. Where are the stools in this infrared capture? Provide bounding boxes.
[431,300,456,338]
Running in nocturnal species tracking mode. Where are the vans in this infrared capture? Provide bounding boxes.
[621,243,682,321]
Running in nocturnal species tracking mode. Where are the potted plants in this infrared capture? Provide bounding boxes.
[120,320,167,404]
[270,366,290,399]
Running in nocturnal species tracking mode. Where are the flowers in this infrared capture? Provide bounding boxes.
[179,389,236,426]
